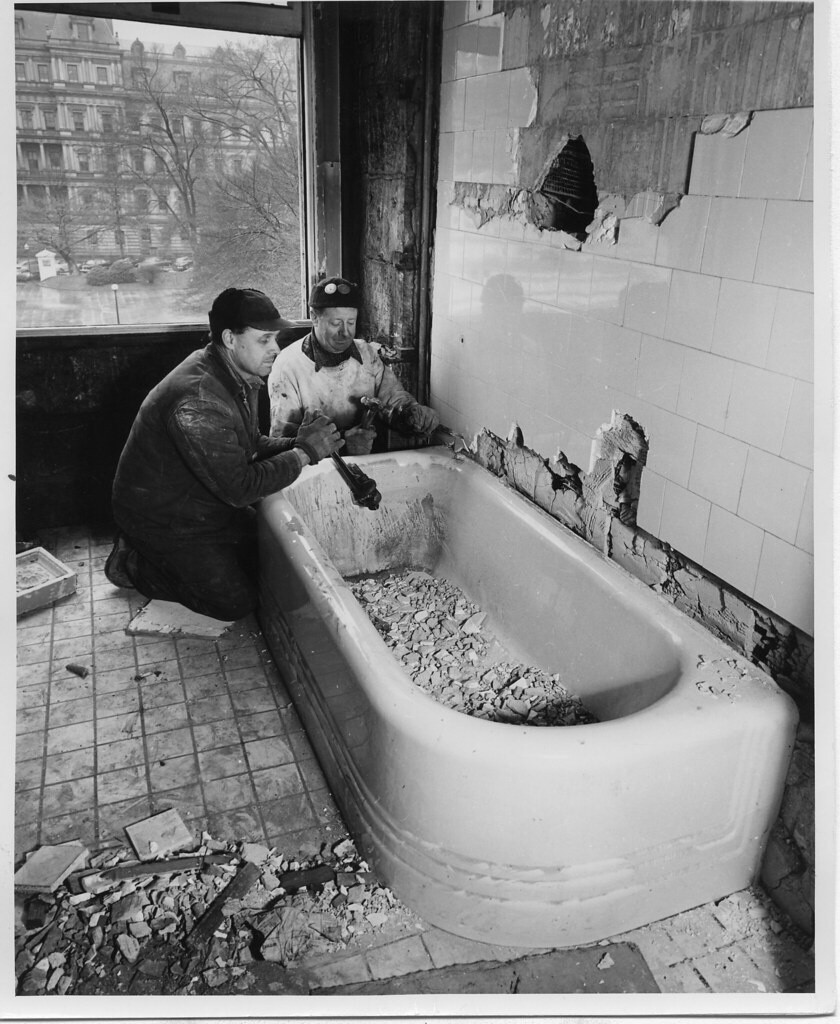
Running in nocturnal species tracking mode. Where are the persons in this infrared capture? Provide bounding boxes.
[263,275,440,457]
[105,286,346,622]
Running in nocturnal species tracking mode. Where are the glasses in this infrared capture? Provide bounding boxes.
[314,283,358,294]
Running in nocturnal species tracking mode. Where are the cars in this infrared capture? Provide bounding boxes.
[18,255,194,280]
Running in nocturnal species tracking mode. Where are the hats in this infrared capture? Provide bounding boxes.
[208,287,295,331]
[309,277,363,308]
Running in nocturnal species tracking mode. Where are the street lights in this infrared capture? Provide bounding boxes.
[111,283,121,325]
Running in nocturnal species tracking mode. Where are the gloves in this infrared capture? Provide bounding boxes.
[291,408,345,466]
[344,424,377,455]
[406,402,440,435]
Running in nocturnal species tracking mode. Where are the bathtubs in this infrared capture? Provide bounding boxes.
[238,445,802,951]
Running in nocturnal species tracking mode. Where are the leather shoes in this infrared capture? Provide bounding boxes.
[104,530,135,588]
